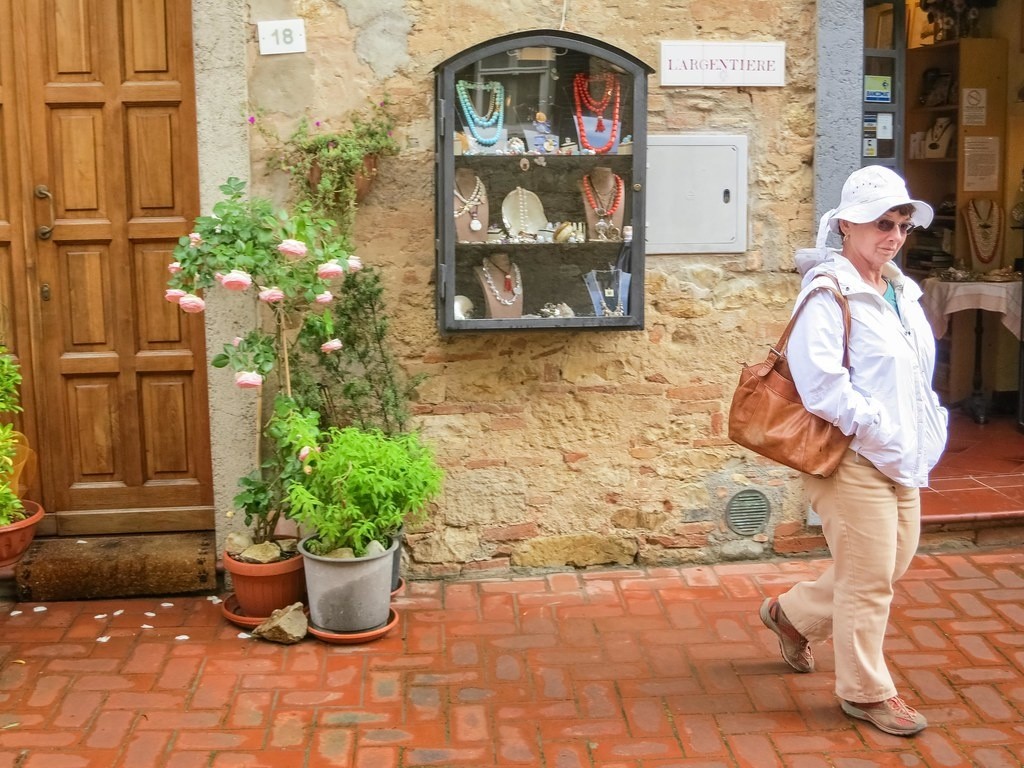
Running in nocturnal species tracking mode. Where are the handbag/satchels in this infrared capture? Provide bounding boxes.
[727,286,856,478]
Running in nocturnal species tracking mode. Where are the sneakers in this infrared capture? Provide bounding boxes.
[759,598,816,674]
[842,696,928,735]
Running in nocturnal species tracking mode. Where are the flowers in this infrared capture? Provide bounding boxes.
[151,87,398,475]
[919,0,980,29]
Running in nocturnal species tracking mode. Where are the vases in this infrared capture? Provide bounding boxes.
[221,517,404,643]
[307,151,378,206]
[941,7,978,41]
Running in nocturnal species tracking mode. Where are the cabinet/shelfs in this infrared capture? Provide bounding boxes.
[436,33,648,333]
[904,37,1011,412]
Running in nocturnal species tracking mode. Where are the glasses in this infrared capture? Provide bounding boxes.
[872,219,915,235]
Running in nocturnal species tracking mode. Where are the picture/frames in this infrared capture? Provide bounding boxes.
[874,3,910,50]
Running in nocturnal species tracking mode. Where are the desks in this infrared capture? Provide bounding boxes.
[920,270,1022,424]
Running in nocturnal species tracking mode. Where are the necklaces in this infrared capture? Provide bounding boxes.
[967,199,1001,263]
[592,269,624,316]
[516,186,530,230]
[573,73,620,153]
[483,256,521,306]
[456,80,506,145]
[453,175,486,232]
[928,121,952,149]
[583,173,622,217]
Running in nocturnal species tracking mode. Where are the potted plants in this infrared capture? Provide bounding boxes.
[0,344,54,568]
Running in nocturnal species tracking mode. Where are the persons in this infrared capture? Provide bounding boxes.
[760,164,949,736]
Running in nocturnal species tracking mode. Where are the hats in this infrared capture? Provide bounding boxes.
[815,165,934,249]
